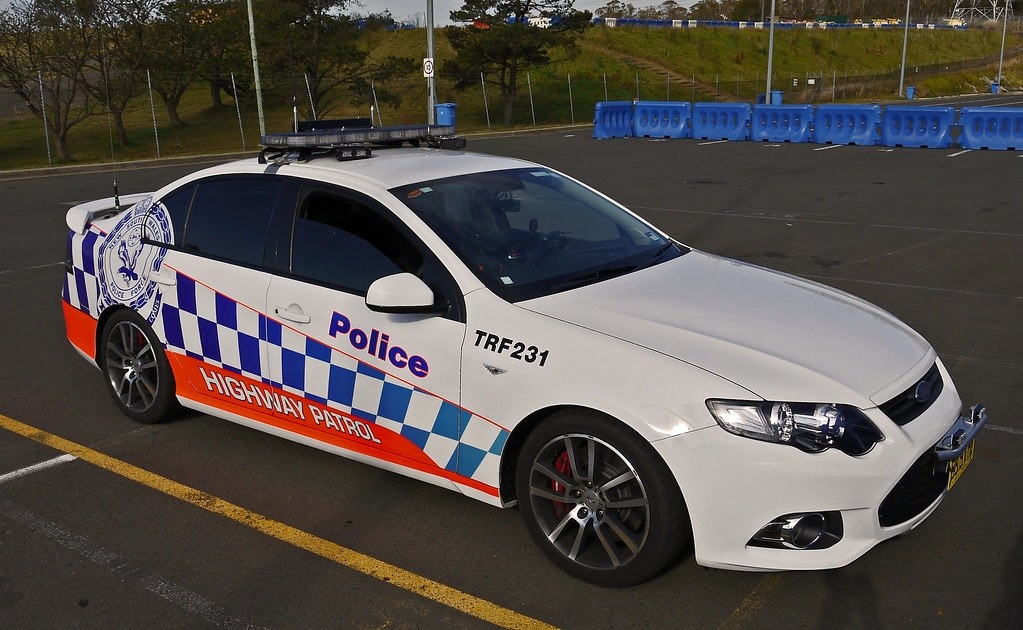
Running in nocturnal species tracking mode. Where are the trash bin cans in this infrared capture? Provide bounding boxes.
[992,83,999,94]
[770,90,785,105]
[433,102,458,125]
[906,86,915,100]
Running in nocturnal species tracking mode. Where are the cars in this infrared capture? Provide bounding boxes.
[59,115,988,587]
